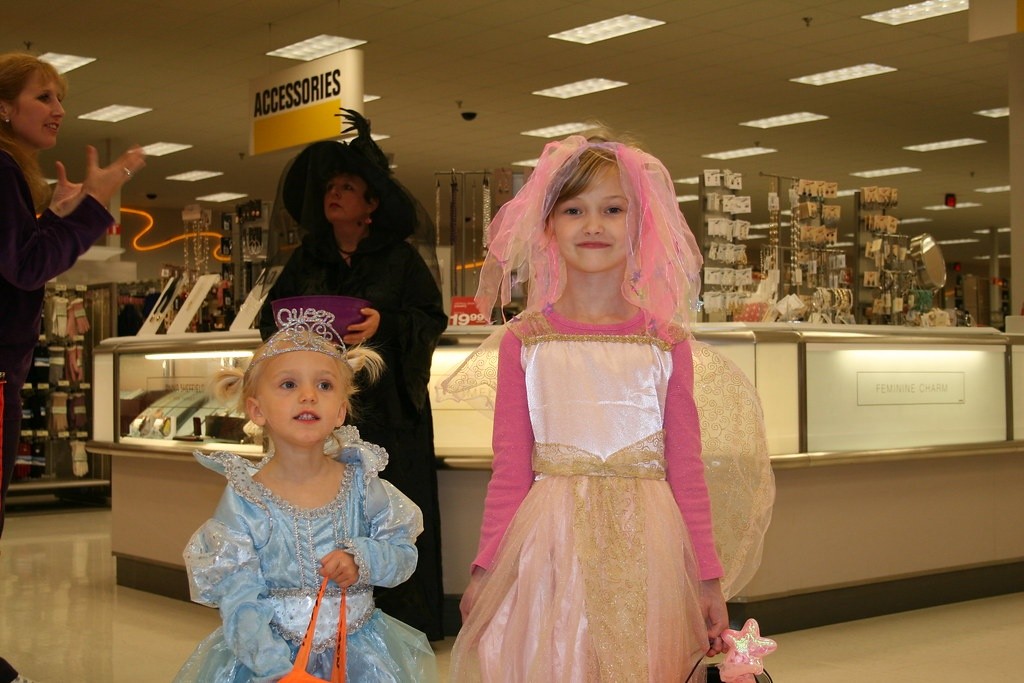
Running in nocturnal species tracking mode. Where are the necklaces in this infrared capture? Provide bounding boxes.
[339,247,356,255]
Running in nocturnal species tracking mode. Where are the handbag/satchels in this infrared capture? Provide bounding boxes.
[276,573,348,683]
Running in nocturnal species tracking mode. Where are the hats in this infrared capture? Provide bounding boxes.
[281,107,419,242]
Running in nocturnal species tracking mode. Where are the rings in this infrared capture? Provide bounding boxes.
[124,167,131,176]
[362,337,367,342]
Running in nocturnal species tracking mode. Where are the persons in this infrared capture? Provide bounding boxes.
[249,107,445,641]
[182,306,438,683]
[449,133,732,683]
[0,51,145,683]
